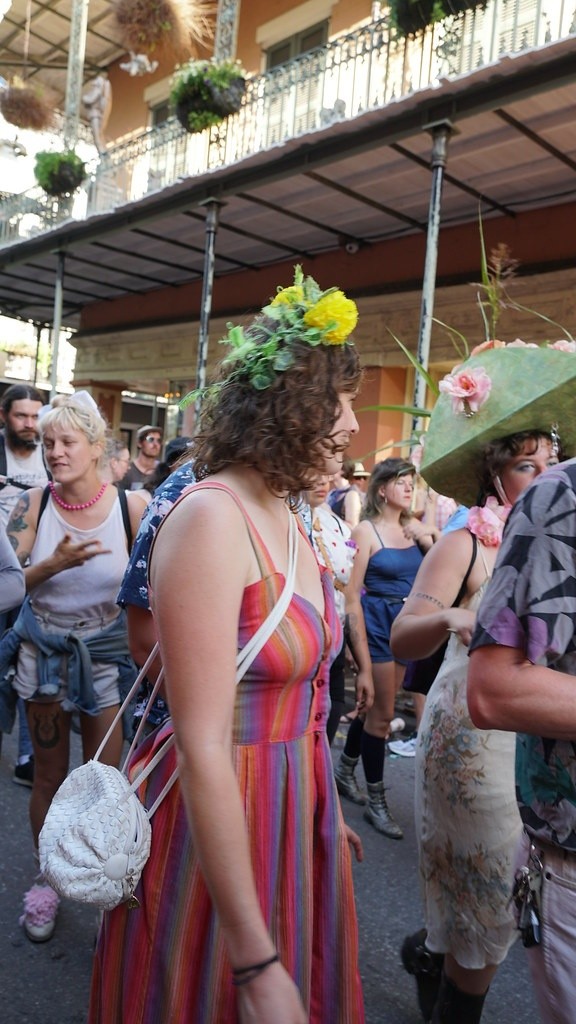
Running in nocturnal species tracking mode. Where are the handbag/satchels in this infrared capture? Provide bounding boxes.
[397,533,478,695]
[38,761,153,908]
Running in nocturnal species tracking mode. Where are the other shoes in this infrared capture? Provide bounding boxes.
[13,755,34,787]
[20,885,59,941]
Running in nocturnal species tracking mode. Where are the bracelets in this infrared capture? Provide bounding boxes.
[231,953,281,986]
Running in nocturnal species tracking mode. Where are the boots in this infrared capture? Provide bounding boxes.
[399,930,442,1024]
[335,751,368,805]
[428,973,490,1024]
[363,781,403,838]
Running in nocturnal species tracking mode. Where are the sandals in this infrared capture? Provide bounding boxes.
[339,712,355,723]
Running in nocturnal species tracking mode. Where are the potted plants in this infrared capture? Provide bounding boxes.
[34,148,92,199]
[384,0,488,36]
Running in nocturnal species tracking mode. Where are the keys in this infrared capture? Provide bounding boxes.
[506,870,543,929]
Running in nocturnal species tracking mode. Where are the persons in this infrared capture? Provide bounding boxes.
[82,72,113,172]
[389,338,575,1024]
[0,384,52,790]
[0,517,26,610]
[87,313,364,1024]
[464,456,576,1024]
[298,454,435,839]
[7,389,148,942]
[101,423,200,500]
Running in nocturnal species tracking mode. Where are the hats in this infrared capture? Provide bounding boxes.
[352,463,371,476]
[136,425,163,449]
[422,340,576,505]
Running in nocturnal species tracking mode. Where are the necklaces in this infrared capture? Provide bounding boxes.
[49,481,108,510]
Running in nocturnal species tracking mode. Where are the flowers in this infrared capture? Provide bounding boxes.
[438,337,576,414]
[220,259,360,389]
[165,53,253,115]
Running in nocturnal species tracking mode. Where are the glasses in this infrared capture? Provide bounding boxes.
[145,436,162,444]
[354,475,366,480]
[119,459,133,466]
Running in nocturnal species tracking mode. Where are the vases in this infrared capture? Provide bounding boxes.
[177,77,247,132]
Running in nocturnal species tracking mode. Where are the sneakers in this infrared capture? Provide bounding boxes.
[388,731,419,756]
